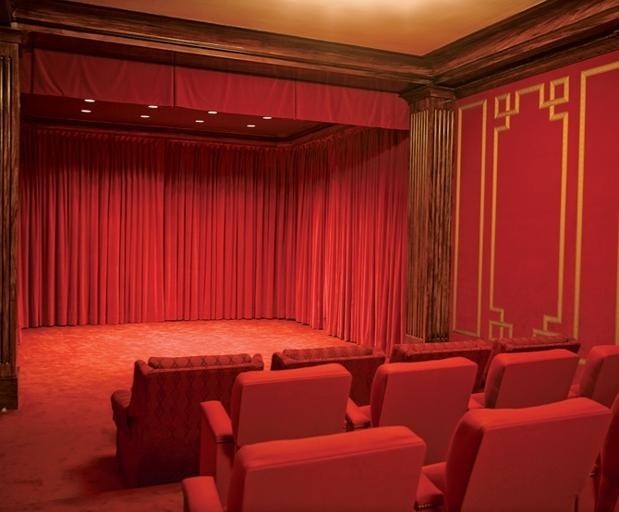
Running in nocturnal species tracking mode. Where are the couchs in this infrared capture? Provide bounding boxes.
[109,352,266,480]
[569,343,619,410]
[416,395,610,511]
[576,392,619,510]
[344,358,481,469]
[199,362,352,506]
[473,345,579,410]
[272,344,385,406]
[181,423,424,511]
[391,339,490,397]
[490,334,582,387]
